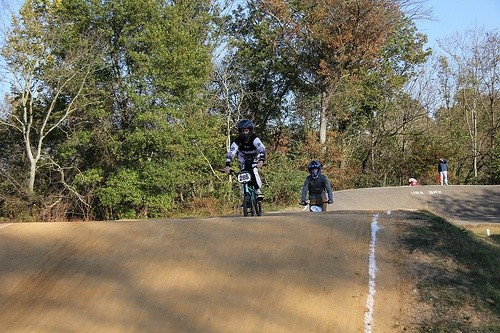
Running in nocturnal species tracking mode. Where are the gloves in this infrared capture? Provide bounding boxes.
[301,201,305,205]
[328,200,333,203]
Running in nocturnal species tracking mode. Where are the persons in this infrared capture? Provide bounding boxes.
[225,120,266,203]
[438,157,448,186]
[301,160,333,213]
[408,178,419,186]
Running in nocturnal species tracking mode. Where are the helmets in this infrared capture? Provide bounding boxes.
[308,161,321,180]
[238,119,253,141]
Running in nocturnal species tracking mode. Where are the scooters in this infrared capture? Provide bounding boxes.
[299,200,331,212]
[218,162,265,216]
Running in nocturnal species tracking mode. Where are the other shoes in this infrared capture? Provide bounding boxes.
[256,189,265,199]
[241,201,250,207]
